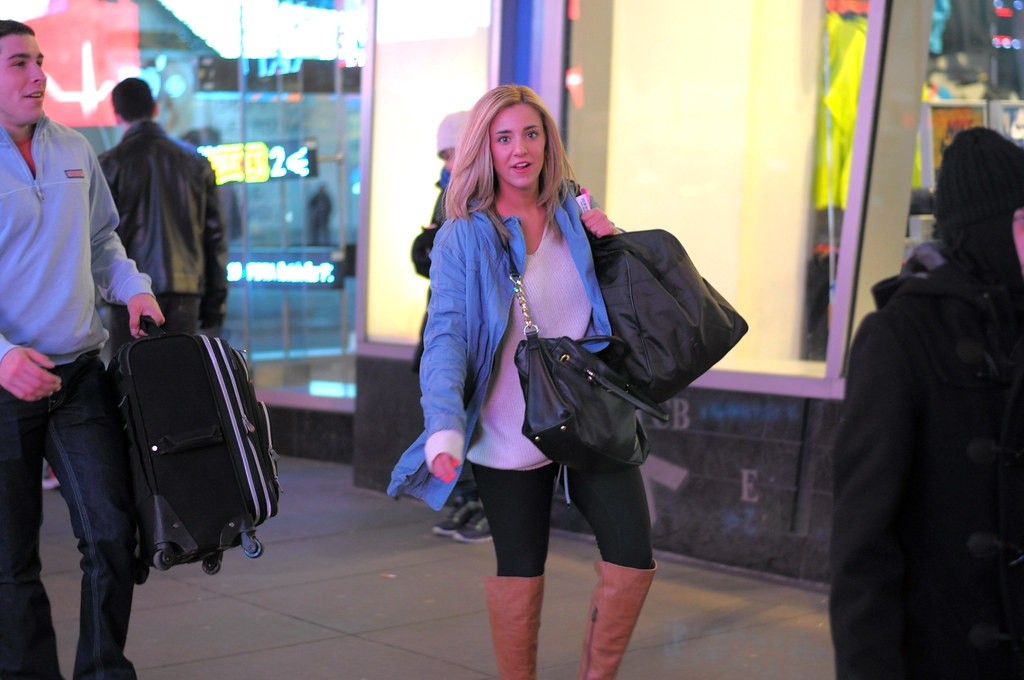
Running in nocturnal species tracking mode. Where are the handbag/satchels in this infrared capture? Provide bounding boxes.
[588,228,749,405]
[509,270,673,476]
[100,313,287,587]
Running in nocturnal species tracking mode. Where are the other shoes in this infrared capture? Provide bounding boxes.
[452,509,494,543]
[431,497,483,543]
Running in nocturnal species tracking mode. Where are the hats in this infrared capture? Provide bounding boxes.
[436,111,472,157]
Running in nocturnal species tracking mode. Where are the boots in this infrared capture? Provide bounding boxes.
[480,575,545,680]
[577,558,658,680]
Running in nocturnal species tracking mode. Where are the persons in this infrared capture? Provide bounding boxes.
[385,81,657,680]
[411,110,492,542]
[96,76,229,361]
[828,125,1024,680]
[0,19,166,680]
